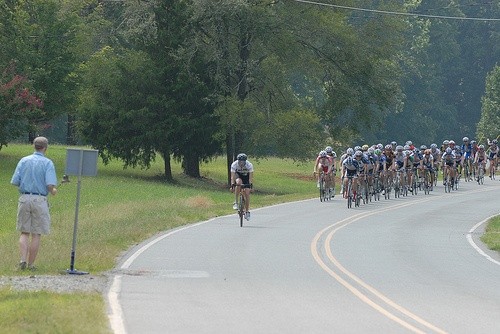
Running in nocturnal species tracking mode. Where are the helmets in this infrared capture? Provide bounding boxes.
[354,146,361,151]
[237,153,247,160]
[363,151,371,156]
[371,136,499,156]
[362,145,368,151]
[346,147,354,155]
[325,146,332,152]
[355,150,363,156]
[368,148,374,153]
[319,150,327,157]
[347,156,353,165]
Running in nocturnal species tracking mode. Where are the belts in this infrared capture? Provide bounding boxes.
[22,192,46,196]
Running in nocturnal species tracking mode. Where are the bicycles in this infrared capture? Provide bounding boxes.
[313,157,498,209]
[231,183,253,227]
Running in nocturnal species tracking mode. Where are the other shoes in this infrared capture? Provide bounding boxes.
[28,264,36,272]
[246,211,250,220]
[233,203,238,210]
[17,261,26,270]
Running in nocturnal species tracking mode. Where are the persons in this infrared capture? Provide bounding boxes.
[313,146,337,197]
[340,137,499,202]
[11,137,57,273]
[230,154,254,220]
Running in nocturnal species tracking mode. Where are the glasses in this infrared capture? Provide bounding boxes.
[321,156,325,158]
[355,155,361,157]
[347,154,352,156]
[327,152,331,153]
